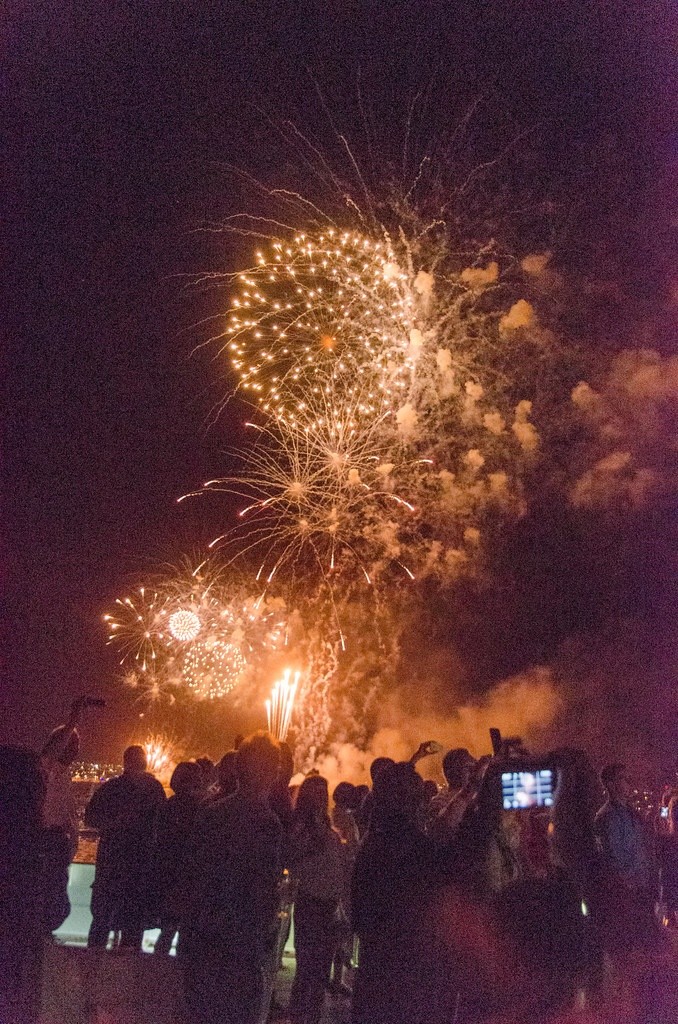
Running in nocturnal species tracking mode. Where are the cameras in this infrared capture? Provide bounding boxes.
[496,762,556,810]
[659,806,670,819]
[430,741,443,751]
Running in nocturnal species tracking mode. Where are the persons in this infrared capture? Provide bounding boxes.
[0,690,678,1024]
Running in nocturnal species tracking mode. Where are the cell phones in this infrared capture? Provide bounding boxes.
[85,698,106,707]
